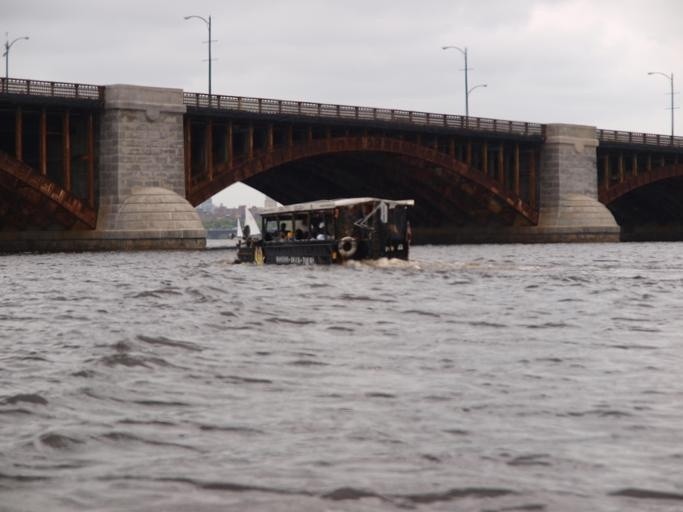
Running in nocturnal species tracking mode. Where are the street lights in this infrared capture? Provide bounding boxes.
[643,67,676,147]
[181,14,214,108]
[3,32,30,91]
[441,42,487,125]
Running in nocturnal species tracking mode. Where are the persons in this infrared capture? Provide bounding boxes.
[278,221,327,241]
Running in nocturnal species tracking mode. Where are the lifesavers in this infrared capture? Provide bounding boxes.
[337,236,357,258]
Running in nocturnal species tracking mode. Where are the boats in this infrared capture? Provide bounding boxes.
[230,198,412,267]
